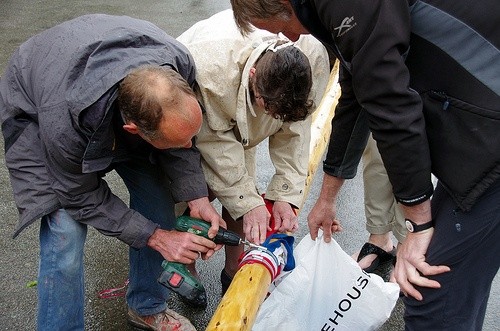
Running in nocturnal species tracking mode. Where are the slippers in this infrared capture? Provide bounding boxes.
[355,241,397,274]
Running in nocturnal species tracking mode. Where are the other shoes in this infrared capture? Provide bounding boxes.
[220,268,233,293]
[178,281,207,312]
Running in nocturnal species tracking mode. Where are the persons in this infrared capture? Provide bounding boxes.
[0,13,227,331]
[231,0,500,331]
[176,8,330,307]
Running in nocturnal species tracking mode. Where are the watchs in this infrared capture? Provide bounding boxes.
[404,218,434,233]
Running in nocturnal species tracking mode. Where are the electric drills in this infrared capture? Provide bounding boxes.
[157,216,250,300]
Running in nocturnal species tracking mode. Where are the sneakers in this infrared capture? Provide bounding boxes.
[128,305,198,331]
